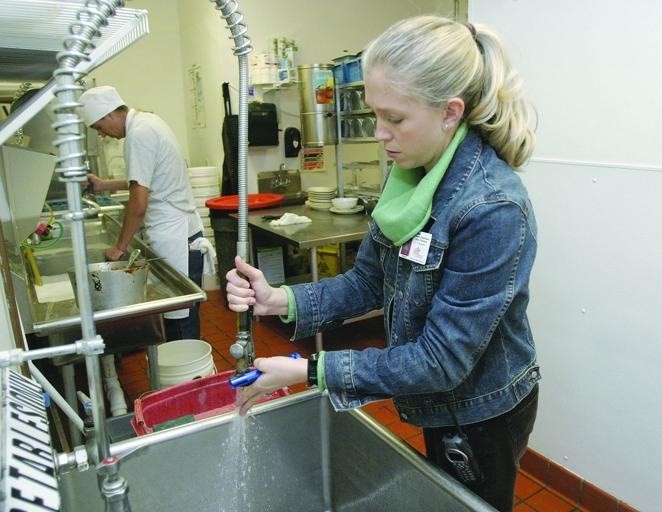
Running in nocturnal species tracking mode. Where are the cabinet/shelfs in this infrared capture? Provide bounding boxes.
[334,57,398,211]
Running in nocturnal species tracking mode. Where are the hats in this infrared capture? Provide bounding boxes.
[77,85,125,128]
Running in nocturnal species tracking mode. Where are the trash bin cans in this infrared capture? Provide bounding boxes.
[131,366,292,436]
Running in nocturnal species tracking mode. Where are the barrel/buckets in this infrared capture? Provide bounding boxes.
[205,193,285,312]
[146,339,217,392]
[297,65,339,147]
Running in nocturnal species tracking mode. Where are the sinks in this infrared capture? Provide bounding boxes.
[56,388,487,512]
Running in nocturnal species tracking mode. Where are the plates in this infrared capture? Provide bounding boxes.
[328,204,365,215]
[304,185,339,212]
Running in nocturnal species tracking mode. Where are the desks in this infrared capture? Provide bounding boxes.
[229,206,382,355]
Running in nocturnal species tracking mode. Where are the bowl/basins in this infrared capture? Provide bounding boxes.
[331,197,359,209]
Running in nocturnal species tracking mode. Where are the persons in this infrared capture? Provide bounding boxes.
[225,14,543,512]
[74,84,217,341]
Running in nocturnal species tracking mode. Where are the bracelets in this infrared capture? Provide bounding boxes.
[305,351,320,392]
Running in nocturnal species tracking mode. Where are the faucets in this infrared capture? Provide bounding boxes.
[52,1,254,470]
